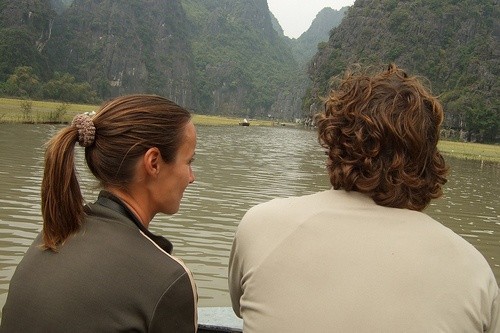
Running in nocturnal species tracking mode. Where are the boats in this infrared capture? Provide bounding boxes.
[239,122,250,126]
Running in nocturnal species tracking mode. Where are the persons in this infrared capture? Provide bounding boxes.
[228,64,500,333]
[0,95,198,333]
